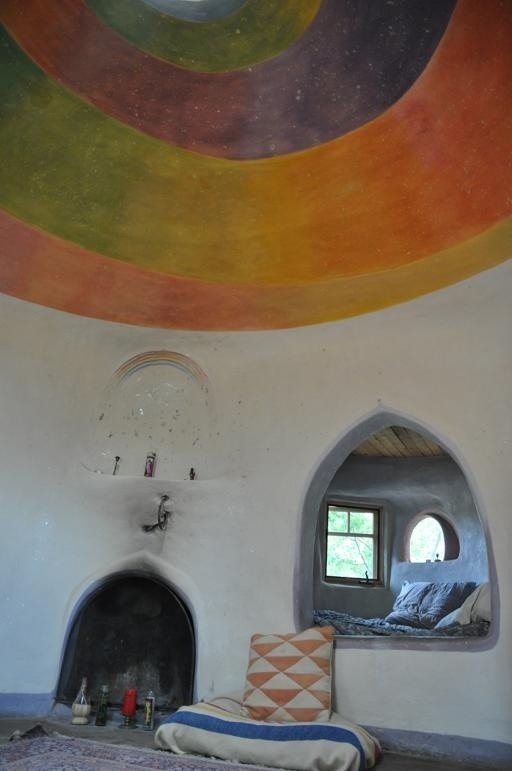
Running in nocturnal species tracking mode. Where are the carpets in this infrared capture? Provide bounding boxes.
[0,732,289,771]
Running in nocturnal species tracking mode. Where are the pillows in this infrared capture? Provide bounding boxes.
[434,581,491,629]
[241,626,335,722]
[385,580,477,628]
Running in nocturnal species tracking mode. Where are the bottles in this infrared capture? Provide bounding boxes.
[72,676,92,726]
[94,684,114,726]
[143,688,156,732]
